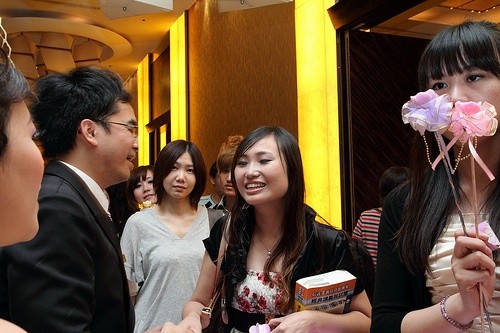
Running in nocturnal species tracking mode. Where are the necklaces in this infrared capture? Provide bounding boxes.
[255,218,281,256]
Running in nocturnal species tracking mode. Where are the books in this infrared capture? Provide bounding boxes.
[293,269,358,315]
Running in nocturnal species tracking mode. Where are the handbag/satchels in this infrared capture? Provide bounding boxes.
[199,211,232,333]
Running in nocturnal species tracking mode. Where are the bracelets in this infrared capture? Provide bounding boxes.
[441,295,474,330]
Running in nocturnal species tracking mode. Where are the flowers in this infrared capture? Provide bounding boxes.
[402,89,498,181]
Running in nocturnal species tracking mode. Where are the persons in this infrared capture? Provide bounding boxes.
[181,125,374,333]
[352,167,413,275]
[116,165,158,238]
[32,130,55,170]
[106,181,132,233]
[212,136,244,216]
[1,66,138,333]
[370,20,499,333]
[202,160,222,211]
[119,140,227,333]
[0,47,195,333]
[104,189,112,212]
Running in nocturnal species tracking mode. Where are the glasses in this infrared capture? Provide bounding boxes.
[78,118,138,137]
